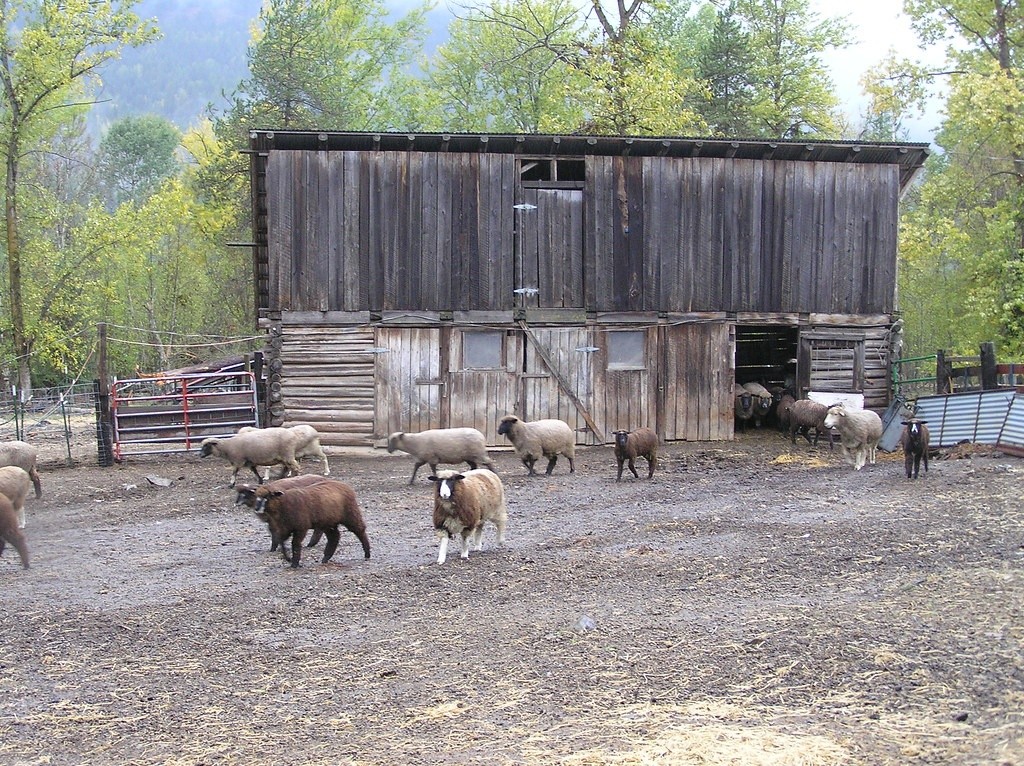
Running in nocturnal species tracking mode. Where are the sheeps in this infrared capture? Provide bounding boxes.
[426,469,506,566]
[0,441,42,569]
[900,419,929,479]
[498,415,575,477]
[735,374,882,470]
[609,428,658,482]
[387,427,495,485]
[199,424,370,568]
